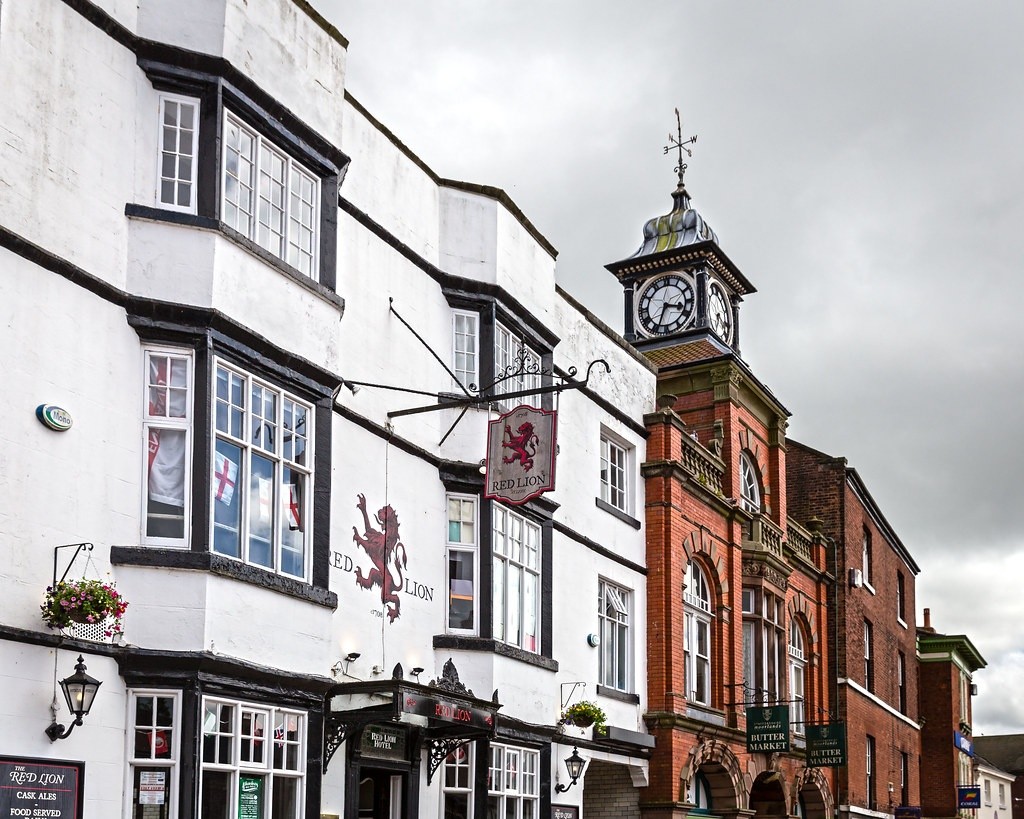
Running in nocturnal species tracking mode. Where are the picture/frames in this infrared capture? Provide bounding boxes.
[0,754,86,819]
[551,803,579,819]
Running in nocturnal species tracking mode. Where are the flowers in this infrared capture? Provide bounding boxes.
[561,700,607,735]
[38,572,129,640]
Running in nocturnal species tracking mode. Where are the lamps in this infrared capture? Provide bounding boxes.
[554,745,586,794]
[410,667,424,676]
[345,653,361,663]
[45,654,103,741]
[970,684,978,695]
[849,567,863,588]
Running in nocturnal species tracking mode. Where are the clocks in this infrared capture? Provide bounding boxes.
[631,265,698,341]
[708,265,736,349]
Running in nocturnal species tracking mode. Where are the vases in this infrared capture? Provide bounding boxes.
[67,604,110,625]
[573,714,594,728]
